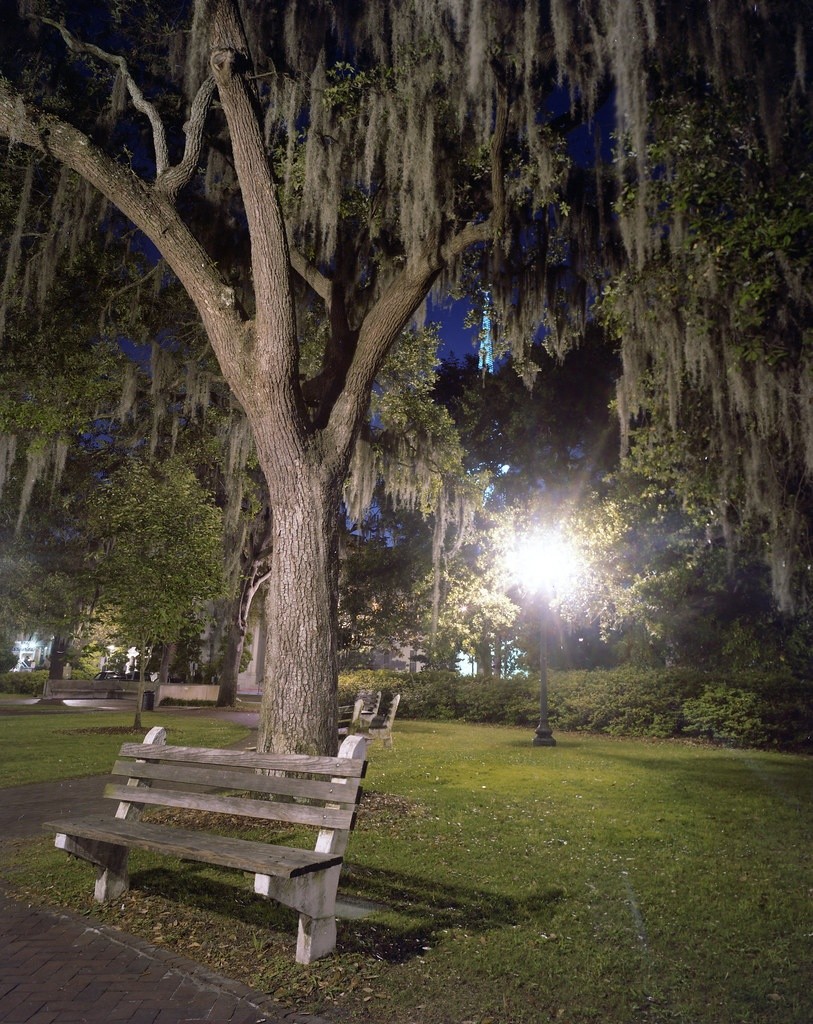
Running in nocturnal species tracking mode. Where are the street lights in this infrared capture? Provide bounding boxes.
[522,529,566,749]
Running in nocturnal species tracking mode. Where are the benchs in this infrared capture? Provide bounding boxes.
[41,726,369,964]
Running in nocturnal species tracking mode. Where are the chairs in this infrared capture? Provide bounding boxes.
[361,691,382,722]
[338,698,365,741]
[363,694,400,753]
